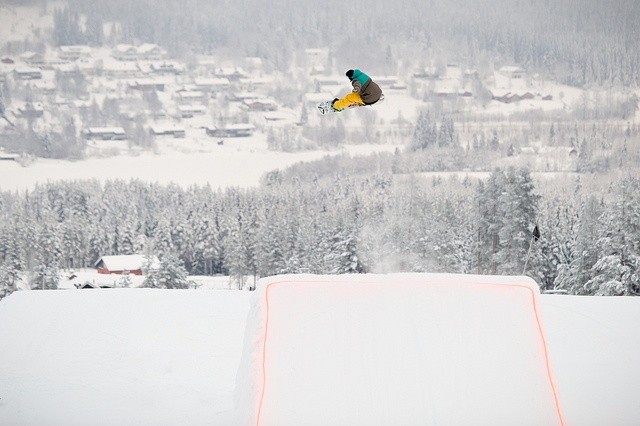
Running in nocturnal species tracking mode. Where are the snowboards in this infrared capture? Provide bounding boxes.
[317,93,385,114]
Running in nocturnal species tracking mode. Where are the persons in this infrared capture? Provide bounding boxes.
[328,68,382,113]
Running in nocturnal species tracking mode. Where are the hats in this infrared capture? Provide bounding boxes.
[345,69,354,81]
